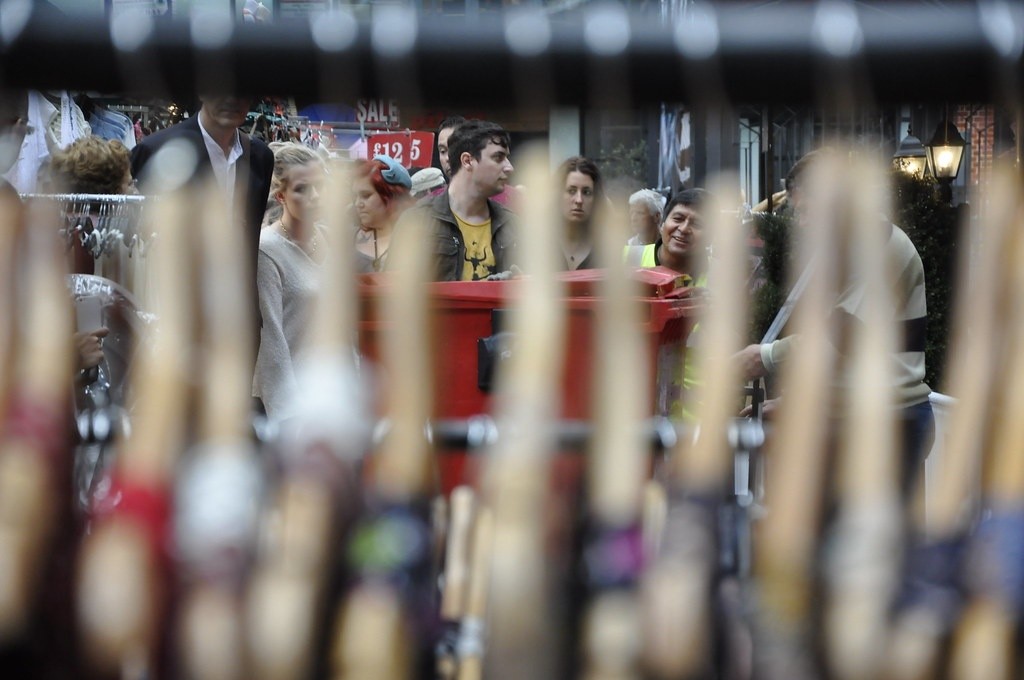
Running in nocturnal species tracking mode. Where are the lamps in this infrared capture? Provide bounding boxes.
[892,124,927,183]
[922,118,971,190]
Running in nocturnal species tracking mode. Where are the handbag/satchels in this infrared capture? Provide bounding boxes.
[476,335,496,392]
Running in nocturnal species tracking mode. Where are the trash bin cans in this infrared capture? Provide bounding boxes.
[346,267,706,555]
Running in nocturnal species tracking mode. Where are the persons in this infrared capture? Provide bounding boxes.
[4,71,936,661]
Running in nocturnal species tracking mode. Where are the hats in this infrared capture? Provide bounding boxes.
[408,167,446,197]
[373,155,412,190]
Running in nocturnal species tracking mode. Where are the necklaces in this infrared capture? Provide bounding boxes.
[560,229,586,265]
[276,214,319,258]
[369,224,397,271]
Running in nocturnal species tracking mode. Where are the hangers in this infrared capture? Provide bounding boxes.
[0,0,1024,680]
